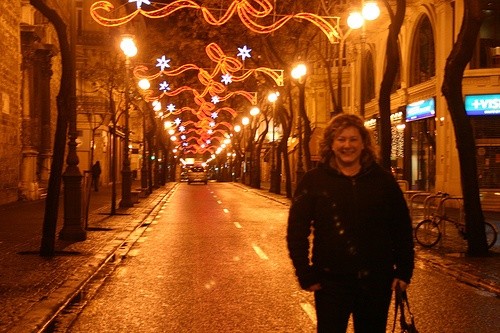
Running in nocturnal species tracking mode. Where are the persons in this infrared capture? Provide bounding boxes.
[91,161,102,192]
[286,112,417,333]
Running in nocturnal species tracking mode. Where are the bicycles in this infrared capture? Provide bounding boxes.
[412,196,497,247]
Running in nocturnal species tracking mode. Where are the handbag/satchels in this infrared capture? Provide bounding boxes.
[392,281,420,333]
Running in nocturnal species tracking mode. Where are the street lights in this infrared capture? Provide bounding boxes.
[346,1,380,118]
[118,33,137,208]
[136,78,152,195]
[290,62,308,184]
[268,91,278,192]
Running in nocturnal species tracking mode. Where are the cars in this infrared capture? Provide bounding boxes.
[186,166,208,184]
